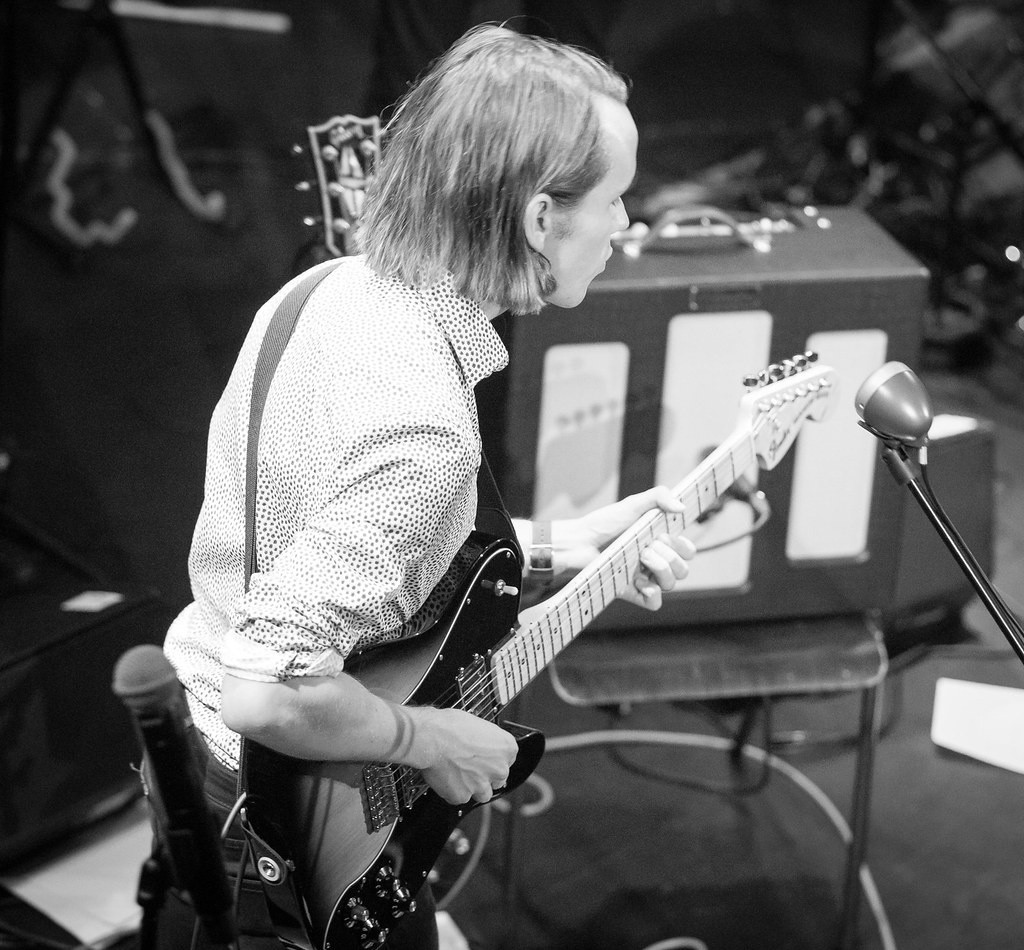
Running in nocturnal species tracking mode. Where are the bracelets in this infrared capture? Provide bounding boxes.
[529,517,556,585]
[381,702,417,764]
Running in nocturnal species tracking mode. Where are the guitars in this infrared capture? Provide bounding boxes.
[239,348,839,950]
[289,115,391,257]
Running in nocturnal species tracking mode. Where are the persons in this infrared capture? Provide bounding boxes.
[160,23,700,950]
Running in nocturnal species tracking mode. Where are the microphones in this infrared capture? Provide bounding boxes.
[113,643,238,946]
[855,360,934,447]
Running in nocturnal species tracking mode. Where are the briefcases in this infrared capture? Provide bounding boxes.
[483,203,929,634]
